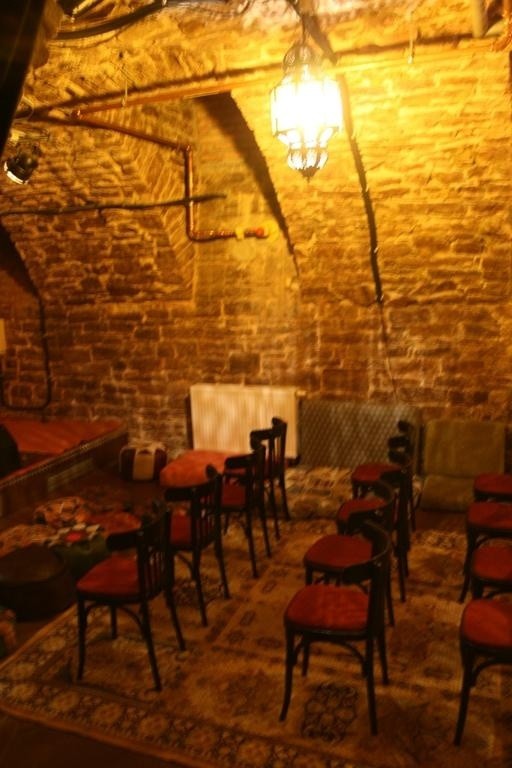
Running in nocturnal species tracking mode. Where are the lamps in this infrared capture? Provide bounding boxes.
[264,14,347,188]
[0,119,56,186]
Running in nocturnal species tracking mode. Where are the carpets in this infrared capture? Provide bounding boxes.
[0,462,510,767]
